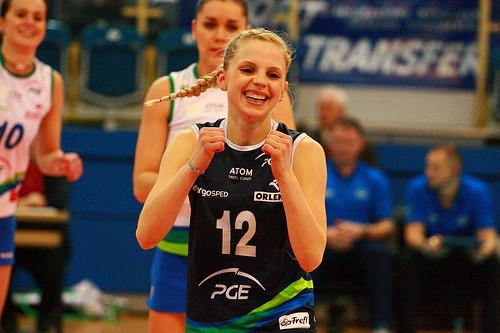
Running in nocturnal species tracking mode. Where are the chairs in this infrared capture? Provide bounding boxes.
[34,19,199,133]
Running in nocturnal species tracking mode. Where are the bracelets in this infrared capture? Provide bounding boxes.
[186,158,206,176]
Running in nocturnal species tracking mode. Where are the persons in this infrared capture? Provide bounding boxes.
[131,2,298,333]
[1,0,83,333]
[400,143,499,333]
[302,84,391,331]
[136,28,330,333]
